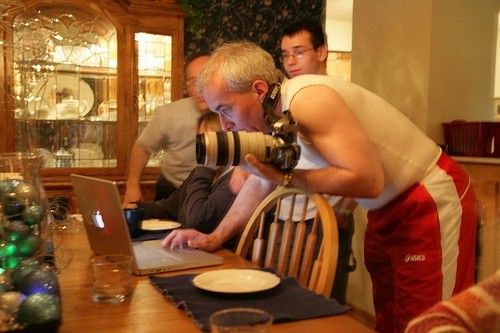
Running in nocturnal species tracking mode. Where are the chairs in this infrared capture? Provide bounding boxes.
[233,186,338,299]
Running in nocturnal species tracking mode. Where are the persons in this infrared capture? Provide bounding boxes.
[161,41,480,333]
[229,20,359,304]
[122,52,243,253]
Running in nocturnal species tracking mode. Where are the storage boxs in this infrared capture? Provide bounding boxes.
[441,119,500,156]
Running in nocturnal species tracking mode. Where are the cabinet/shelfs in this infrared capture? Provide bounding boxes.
[13,64,174,175]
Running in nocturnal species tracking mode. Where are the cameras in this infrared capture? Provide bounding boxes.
[195,116,302,172]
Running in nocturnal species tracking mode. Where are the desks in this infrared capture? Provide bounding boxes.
[50,205,380,333]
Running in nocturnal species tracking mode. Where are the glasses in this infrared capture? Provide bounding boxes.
[280,46,315,62]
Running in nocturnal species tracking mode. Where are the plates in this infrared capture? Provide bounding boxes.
[192,269,282,294]
[98,101,118,121]
[33,75,94,117]
[138,219,182,230]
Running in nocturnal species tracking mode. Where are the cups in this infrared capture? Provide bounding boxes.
[209,308,273,333]
[61,96,87,116]
[123,208,143,239]
[35,97,51,117]
[91,253,133,303]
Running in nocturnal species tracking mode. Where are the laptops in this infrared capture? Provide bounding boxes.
[70,172,224,275]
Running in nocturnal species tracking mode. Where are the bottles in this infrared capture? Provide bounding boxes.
[0,152,48,233]
